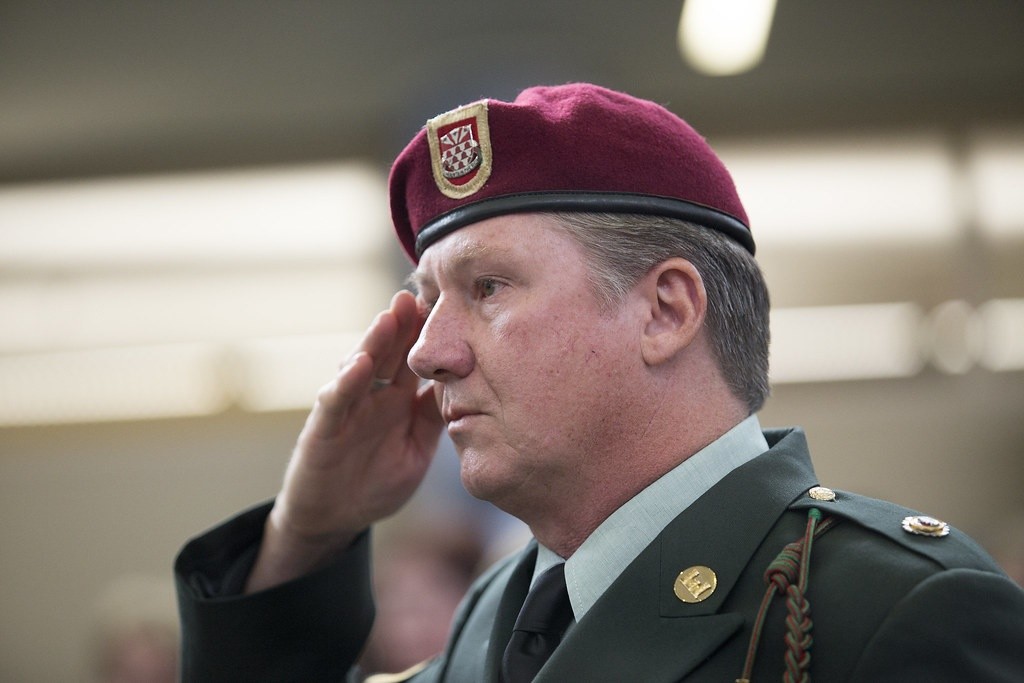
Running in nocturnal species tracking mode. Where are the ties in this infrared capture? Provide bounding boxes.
[497,563,575,683]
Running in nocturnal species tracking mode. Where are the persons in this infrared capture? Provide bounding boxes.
[172,81,1024,683]
[92,407,526,683]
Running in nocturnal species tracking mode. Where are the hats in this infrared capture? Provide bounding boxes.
[388,83,755,256]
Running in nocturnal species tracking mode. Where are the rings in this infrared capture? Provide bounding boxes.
[375,378,393,385]
